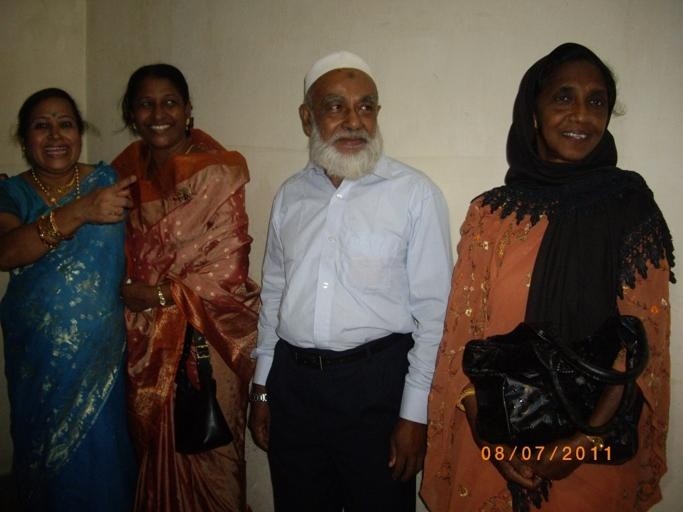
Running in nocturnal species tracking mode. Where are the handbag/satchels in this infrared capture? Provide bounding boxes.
[171,374,234,455]
[461,313,648,467]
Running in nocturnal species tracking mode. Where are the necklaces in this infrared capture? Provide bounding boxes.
[31,163,80,207]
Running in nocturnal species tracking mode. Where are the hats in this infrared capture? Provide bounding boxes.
[304,51,378,97]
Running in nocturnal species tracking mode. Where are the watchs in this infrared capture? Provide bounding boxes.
[156,285,167,306]
[585,435,605,451]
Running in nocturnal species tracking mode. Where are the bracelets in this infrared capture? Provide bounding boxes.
[249,392,268,402]
[456,387,476,412]
[38,209,64,249]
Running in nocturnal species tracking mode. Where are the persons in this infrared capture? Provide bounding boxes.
[419,42,677,512]
[0,87,140,512]
[247,51,454,512]
[111,64,262,512]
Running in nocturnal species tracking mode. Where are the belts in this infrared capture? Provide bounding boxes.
[283,338,400,375]
